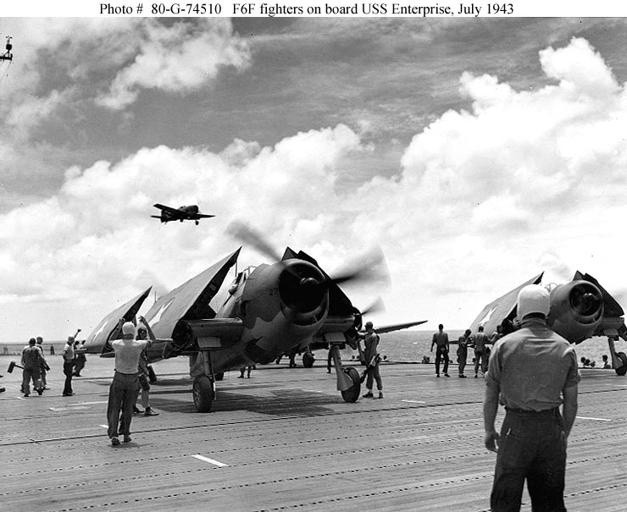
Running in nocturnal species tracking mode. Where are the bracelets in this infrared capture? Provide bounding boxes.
[115,327,121,331]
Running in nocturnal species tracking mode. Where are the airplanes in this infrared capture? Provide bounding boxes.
[448,267,627,379]
[64,221,430,410]
[149,201,216,230]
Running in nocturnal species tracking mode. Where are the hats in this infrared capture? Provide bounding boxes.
[121,320,137,336]
[517,283,552,322]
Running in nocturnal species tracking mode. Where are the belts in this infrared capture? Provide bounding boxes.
[506,406,558,420]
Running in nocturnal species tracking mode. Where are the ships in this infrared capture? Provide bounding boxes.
[0,319,627,510]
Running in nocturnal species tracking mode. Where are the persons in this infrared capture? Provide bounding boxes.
[430,319,519,378]
[581,353,613,369]
[105,315,155,445]
[238,367,252,378]
[21,329,87,395]
[133,328,159,416]
[363,321,384,398]
[484,285,582,512]
[285,352,301,368]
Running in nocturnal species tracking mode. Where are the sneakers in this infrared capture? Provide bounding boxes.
[435,374,479,378]
[363,392,384,399]
[130,406,159,416]
[111,431,132,444]
